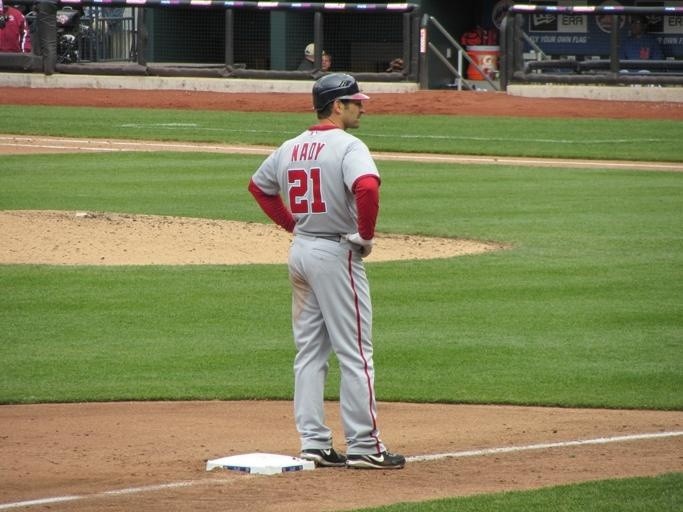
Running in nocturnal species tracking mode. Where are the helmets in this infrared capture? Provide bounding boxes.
[313,72,371,111]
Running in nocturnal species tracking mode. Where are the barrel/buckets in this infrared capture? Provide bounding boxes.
[465,45,502,80]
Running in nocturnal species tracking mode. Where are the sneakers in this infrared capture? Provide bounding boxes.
[346,450,406,469]
[300,447,347,466]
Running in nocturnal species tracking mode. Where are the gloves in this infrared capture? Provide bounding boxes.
[342,232,374,257]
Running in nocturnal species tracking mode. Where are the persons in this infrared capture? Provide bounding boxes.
[248,73,407,469]
[296,44,326,73]
[620,14,665,71]
[386,59,404,73]
[320,53,333,70]
[0,0,126,77]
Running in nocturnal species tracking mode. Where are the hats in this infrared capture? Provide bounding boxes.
[305,43,324,56]
